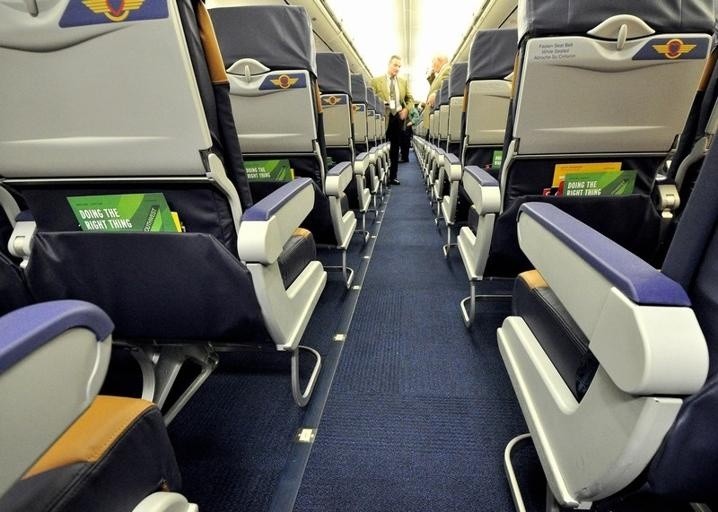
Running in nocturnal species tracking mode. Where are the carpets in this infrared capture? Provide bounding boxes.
[389,77,397,117]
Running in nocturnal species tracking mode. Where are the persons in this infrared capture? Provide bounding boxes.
[371,54,415,185]
[417,103,426,114]
[399,107,420,163]
[423,54,452,142]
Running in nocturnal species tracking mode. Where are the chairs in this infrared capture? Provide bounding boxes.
[3,2,329,408]
[1,297,200,511]
[411,26,519,260]
[497,122,717,512]
[457,2,717,328]
[0,0,394,288]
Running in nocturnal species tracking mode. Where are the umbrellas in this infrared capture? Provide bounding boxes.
[398,159,409,163]
[390,178,400,185]
